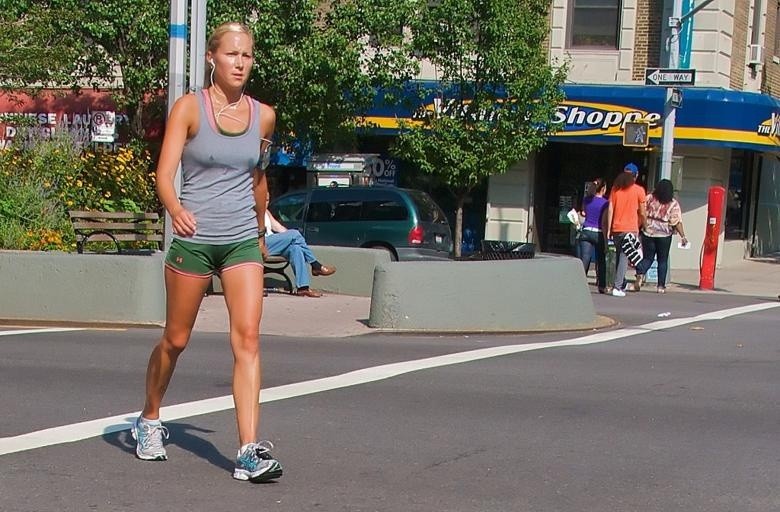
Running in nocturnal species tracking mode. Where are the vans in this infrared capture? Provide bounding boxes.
[266,183,453,263]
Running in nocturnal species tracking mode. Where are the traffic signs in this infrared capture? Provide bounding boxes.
[644,67,696,87]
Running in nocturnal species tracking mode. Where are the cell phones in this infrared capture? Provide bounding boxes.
[260,142,273,170]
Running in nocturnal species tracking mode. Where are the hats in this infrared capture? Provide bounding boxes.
[625,164,638,173]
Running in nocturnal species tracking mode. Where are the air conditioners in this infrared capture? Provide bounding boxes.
[750,44,765,66]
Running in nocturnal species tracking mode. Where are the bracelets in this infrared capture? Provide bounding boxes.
[258,227,267,239]
[681,236,687,239]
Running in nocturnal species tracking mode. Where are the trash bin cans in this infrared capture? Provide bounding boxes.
[481,241,536,259]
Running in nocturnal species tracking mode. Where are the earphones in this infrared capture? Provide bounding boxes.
[210,58,216,68]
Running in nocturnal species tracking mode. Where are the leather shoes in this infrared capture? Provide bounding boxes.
[297,288,321,297]
[312,265,336,276]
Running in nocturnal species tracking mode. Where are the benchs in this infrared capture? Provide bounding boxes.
[65,210,165,254]
[263,256,296,296]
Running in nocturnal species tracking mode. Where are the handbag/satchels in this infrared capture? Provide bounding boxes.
[605,239,617,285]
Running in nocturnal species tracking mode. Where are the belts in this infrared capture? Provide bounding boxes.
[582,227,603,233]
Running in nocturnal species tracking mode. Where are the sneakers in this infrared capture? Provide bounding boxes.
[598,275,664,296]
[234,445,282,481]
[130,415,168,460]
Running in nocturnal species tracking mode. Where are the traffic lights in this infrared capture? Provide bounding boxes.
[622,120,650,149]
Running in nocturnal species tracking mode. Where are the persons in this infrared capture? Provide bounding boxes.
[264,191,337,297]
[634,179,688,293]
[131,23,283,481]
[330,181,338,188]
[607,164,648,296]
[579,178,610,293]
